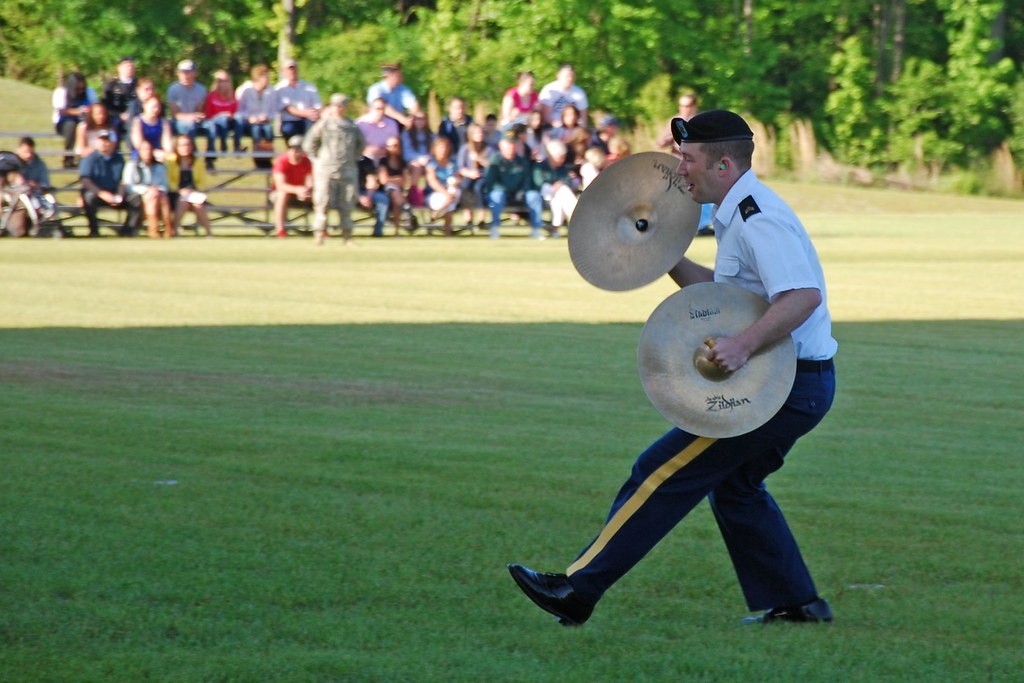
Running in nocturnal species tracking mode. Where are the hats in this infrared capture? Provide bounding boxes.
[285,59,299,69]
[381,62,402,71]
[176,59,196,72]
[326,92,351,107]
[670,110,754,146]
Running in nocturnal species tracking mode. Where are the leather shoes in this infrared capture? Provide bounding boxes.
[508,563,595,628]
[743,597,833,625]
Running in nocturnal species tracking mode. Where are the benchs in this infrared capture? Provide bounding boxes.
[0,133,533,238]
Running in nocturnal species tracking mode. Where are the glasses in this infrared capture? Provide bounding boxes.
[373,106,385,111]
[288,145,301,150]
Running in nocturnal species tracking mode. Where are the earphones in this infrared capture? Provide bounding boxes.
[721,163,726,169]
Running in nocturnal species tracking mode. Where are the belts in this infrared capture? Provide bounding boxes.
[797,357,833,372]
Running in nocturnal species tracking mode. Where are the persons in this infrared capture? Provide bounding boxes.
[0,59,714,244]
[505,109,837,629]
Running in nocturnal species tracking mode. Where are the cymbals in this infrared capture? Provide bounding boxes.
[636,282,797,437]
[566,151,703,293]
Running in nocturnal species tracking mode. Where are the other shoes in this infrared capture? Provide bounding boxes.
[529,229,547,242]
[490,226,501,240]
[64,157,78,169]
